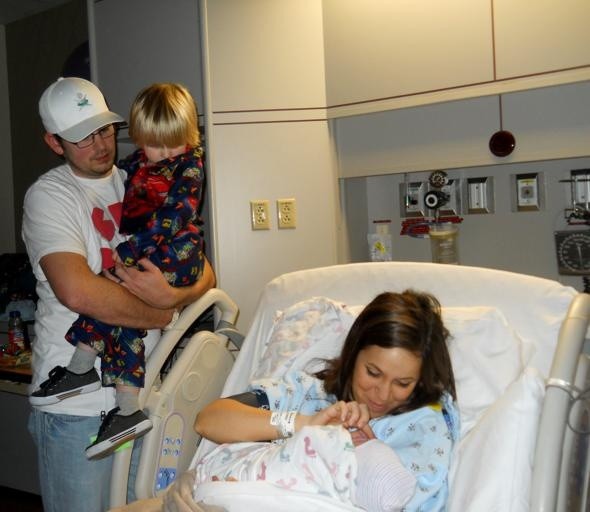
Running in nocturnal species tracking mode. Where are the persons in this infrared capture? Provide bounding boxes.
[19,74,216,511]
[188,423,417,511]
[28,82,212,459]
[107,289,459,511]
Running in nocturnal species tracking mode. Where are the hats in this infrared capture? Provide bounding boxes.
[38,77,128,145]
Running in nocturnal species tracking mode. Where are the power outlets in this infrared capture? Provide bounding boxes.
[250,198,272,231]
[276,197,297,231]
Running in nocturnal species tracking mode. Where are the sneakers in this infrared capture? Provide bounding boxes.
[85,408,153,460]
[28,366,101,406]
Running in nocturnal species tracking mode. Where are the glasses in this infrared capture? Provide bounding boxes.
[53,122,118,148]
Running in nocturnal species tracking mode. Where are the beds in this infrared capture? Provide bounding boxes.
[105,256,590,511]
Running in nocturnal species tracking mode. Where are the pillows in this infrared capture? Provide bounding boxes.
[250,293,538,439]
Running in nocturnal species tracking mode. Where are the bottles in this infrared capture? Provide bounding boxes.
[427,218,460,266]
[7,311,25,351]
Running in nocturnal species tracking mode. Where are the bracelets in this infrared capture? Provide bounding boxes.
[270,410,296,439]
[162,308,179,332]
